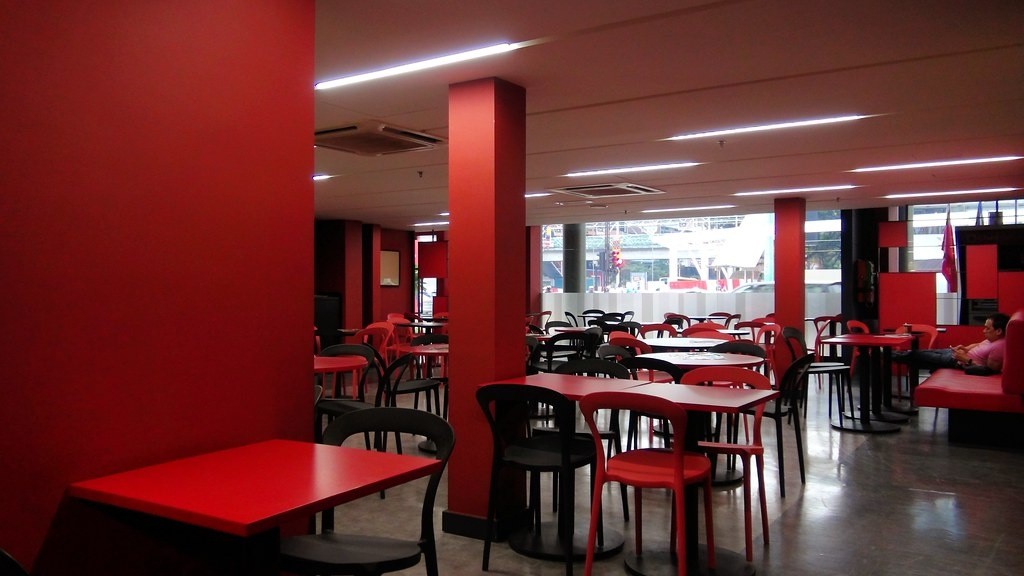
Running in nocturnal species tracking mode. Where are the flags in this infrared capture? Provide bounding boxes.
[943,218,957,292]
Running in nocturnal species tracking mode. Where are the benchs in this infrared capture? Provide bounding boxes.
[916,311,1024,452]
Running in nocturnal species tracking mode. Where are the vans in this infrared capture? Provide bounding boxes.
[727,281,842,293]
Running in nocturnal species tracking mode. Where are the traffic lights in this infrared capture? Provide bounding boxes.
[596,252,604,270]
[608,250,622,272]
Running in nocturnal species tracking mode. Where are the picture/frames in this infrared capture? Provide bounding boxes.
[380,249,400,286]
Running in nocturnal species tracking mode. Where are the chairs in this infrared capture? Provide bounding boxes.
[314,314,937,576]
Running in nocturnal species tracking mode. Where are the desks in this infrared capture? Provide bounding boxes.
[69,316,918,576]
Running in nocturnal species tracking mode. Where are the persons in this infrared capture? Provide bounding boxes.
[878,312,1010,375]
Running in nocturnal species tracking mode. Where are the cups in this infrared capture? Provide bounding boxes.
[904,324,912,334]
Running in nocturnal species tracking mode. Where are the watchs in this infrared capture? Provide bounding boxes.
[964,359,972,367]
